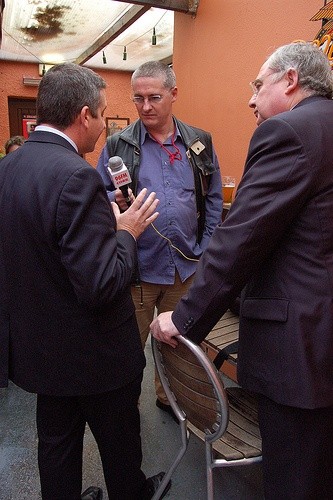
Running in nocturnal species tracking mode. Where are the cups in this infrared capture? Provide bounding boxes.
[221,176,236,205]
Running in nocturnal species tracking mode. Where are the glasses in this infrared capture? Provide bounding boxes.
[129,91,161,106]
[250,71,281,93]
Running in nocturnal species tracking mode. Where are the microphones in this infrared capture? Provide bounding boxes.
[107,156,131,207]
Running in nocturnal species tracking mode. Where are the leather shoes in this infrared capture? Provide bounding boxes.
[82,486,103,500]
[143,472,171,500]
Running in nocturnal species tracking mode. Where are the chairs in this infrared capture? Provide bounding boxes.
[153,334,264,500]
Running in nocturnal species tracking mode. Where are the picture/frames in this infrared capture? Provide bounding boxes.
[105,117,130,138]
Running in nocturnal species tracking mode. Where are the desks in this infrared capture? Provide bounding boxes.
[202,307,240,383]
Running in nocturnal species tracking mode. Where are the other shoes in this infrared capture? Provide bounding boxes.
[155,398,179,425]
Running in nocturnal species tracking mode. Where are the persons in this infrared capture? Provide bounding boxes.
[0,61,171,500]
[95,61,223,419]
[5,135,26,156]
[148,39,333,500]
